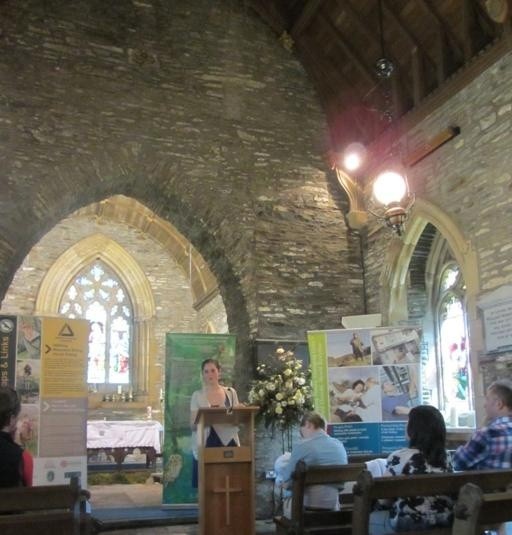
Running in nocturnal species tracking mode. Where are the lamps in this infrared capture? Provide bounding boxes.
[367,171,416,236]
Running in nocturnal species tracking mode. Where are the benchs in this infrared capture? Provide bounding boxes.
[0,476,91,535]
[351,468,512,535]
[273,459,390,535]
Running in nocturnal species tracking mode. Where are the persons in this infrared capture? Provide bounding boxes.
[343,378,380,423]
[274,412,348,520]
[350,333,365,363]
[189,359,246,487]
[367,405,453,534]
[450,378,512,525]
[0,385,33,488]
[382,367,416,421]
[332,380,364,421]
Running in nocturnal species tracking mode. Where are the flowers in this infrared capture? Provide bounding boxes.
[246,342,314,454]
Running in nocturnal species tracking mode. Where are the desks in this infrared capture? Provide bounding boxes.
[87,420,165,471]
